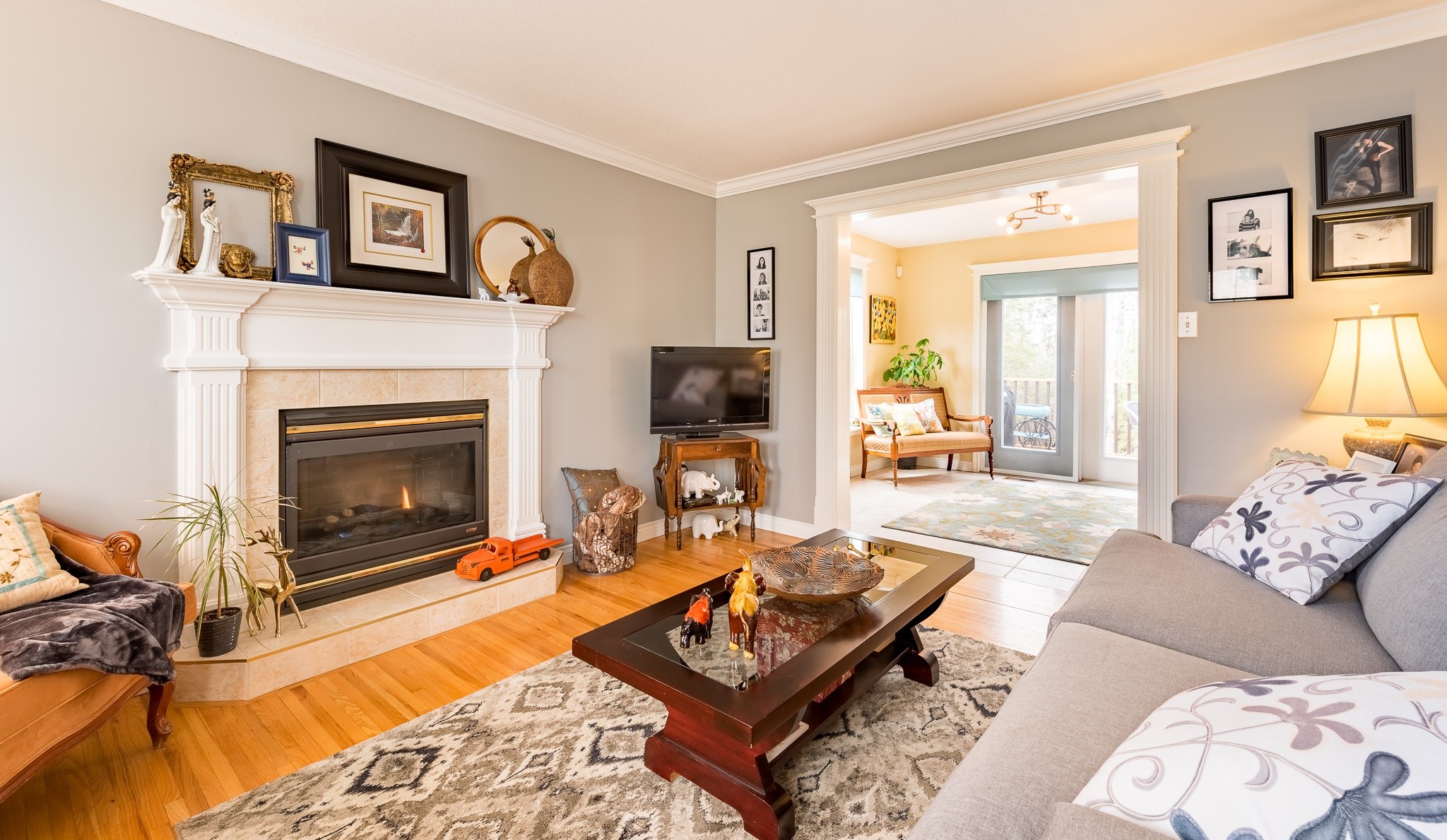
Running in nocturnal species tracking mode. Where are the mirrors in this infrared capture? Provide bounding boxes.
[474,215,548,302]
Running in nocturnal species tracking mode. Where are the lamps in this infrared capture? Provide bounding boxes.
[997,191,1080,235]
[1300,303,1447,459]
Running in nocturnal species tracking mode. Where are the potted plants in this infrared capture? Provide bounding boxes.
[134,456,300,660]
[883,339,946,469]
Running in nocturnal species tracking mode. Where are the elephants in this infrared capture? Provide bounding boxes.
[717,514,741,537]
[724,548,768,659]
[679,587,714,650]
[691,512,723,539]
[716,485,745,505]
[680,470,721,498]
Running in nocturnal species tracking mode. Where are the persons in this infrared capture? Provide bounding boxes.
[1345,135,1394,194]
[753,257,770,333]
[1239,209,1262,257]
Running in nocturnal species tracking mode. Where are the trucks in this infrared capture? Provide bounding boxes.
[454,533,564,582]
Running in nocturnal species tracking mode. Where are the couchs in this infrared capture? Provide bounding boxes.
[1045,445,1447,678]
[910,622,1267,840]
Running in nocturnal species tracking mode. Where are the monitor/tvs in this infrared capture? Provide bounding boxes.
[647,346,771,436]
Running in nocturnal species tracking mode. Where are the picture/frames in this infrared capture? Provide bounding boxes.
[1206,114,1433,304]
[747,247,775,340]
[1267,432,1447,476]
[167,138,472,299]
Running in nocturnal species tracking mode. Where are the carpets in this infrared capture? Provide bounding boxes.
[882,480,1139,567]
[172,623,1036,840]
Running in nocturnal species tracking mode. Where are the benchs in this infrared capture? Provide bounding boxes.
[0,517,198,805]
[857,383,994,489]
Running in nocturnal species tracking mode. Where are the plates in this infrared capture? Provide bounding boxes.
[746,546,884,601]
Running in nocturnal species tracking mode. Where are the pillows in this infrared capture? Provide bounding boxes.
[1191,453,1447,606]
[865,397,945,437]
[562,467,621,513]
[0,491,90,613]
[669,366,725,405]
[1073,670,1446,840]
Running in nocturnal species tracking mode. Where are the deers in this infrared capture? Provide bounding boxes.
[238,523,307,638]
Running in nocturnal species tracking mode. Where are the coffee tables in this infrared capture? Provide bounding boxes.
[573,528,976,839]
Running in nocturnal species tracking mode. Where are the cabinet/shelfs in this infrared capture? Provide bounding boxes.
[653,431,767,550]
